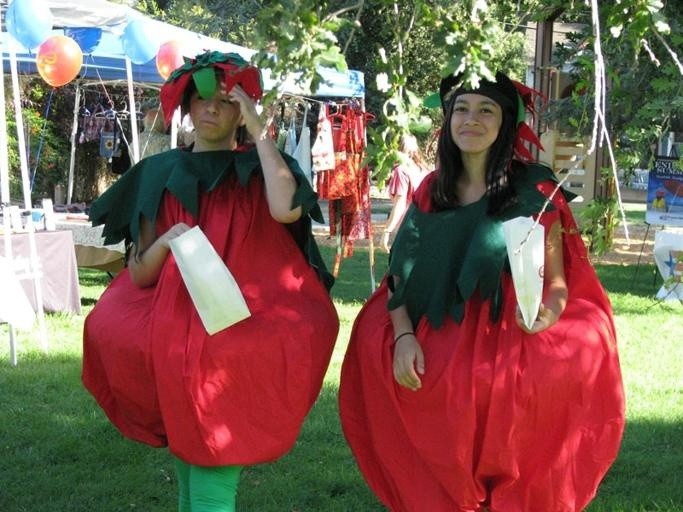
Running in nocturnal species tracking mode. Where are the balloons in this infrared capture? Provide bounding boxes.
[63,25,102,54]
[156,38,195,83]
[36,34,84,92]
[122,16,169,65]
[4,0,53,51]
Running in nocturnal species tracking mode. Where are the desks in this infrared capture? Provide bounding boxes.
[41,214,130,279]
[0,223,85,318]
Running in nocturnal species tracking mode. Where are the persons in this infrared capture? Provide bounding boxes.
[126,106,172,167]
[553,81,586,202]
[336,71,627,512]
[377,132,431,255]
[78,47,341,512]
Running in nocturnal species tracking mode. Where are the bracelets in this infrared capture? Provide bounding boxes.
[392,331,415,346]
[382,228,392,234]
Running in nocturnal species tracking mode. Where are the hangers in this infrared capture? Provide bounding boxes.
[268,92,379,139]
[67,87,161,124]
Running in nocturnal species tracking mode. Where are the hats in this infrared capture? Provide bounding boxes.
[439,66,521,127]
